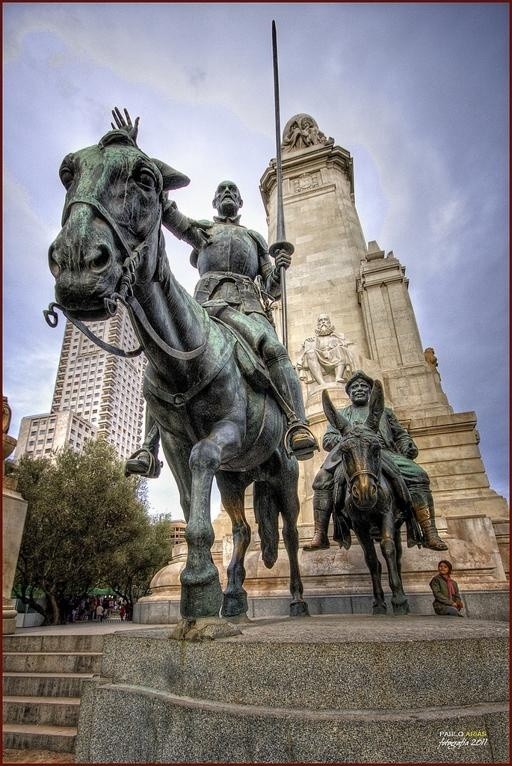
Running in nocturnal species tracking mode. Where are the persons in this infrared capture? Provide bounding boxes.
[109,105,317,478]
[297,312,362,384]
[71,594,131,622]
[426,560,464,617]
[302,370,449,553]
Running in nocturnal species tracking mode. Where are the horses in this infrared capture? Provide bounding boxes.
[47,129,311,620]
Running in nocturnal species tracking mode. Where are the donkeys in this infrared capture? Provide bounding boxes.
[322,379,409,615]
